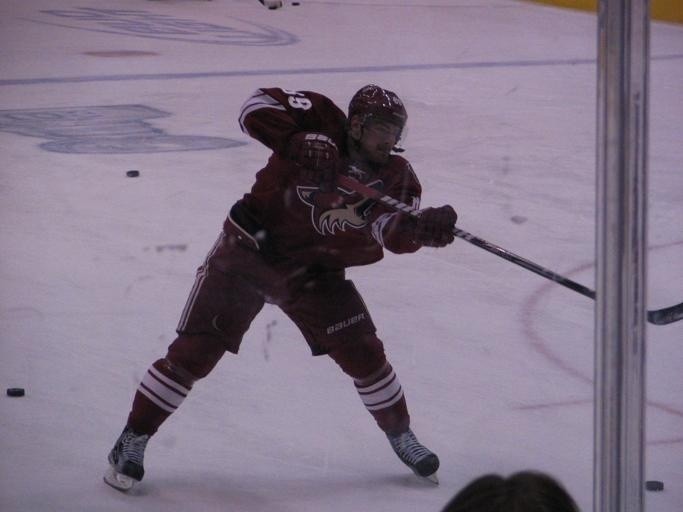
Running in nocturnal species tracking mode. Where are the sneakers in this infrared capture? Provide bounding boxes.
[107,426,150,481]
[388,429,439,477]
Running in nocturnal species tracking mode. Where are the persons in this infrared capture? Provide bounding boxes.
[104,83,459,482]
[438,468,581,512]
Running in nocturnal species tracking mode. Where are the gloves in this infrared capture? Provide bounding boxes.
[413,205,457,247]
[292,130,340,193]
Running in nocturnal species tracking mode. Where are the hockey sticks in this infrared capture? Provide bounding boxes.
[334,171,683,324]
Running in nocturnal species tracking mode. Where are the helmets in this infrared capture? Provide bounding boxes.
[349,83,408,126]
